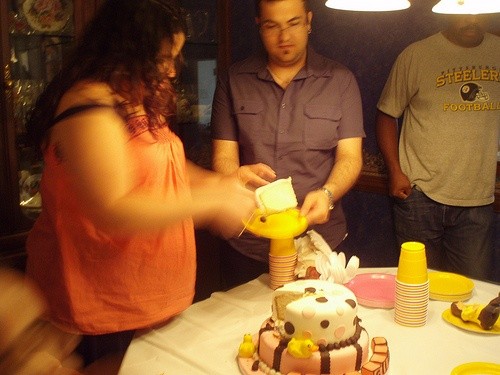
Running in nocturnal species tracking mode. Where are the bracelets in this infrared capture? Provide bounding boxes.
[316,186,335,212]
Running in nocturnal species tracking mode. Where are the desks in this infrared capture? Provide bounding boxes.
[116,268,500,375]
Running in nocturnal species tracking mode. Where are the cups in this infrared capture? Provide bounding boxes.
[269,237,298,291]
[393,242,429,328]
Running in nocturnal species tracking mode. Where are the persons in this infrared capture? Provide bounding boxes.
[0,269,87,375]
[374,13,500,282]
[22,0,277,364]
[210,0,366,293]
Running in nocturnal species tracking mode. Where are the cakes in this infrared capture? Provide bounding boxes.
[254,177,298,215]
[252,279,370,375]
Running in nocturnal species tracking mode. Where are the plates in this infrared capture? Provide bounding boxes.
[442,307,500,335]
[344,272,395,310]
[450,361,500,375]
[16,0,74,35]
[242,208,308,238]
[427,270,474,302]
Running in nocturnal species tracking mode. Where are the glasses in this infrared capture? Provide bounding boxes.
[263,21,308,31]
